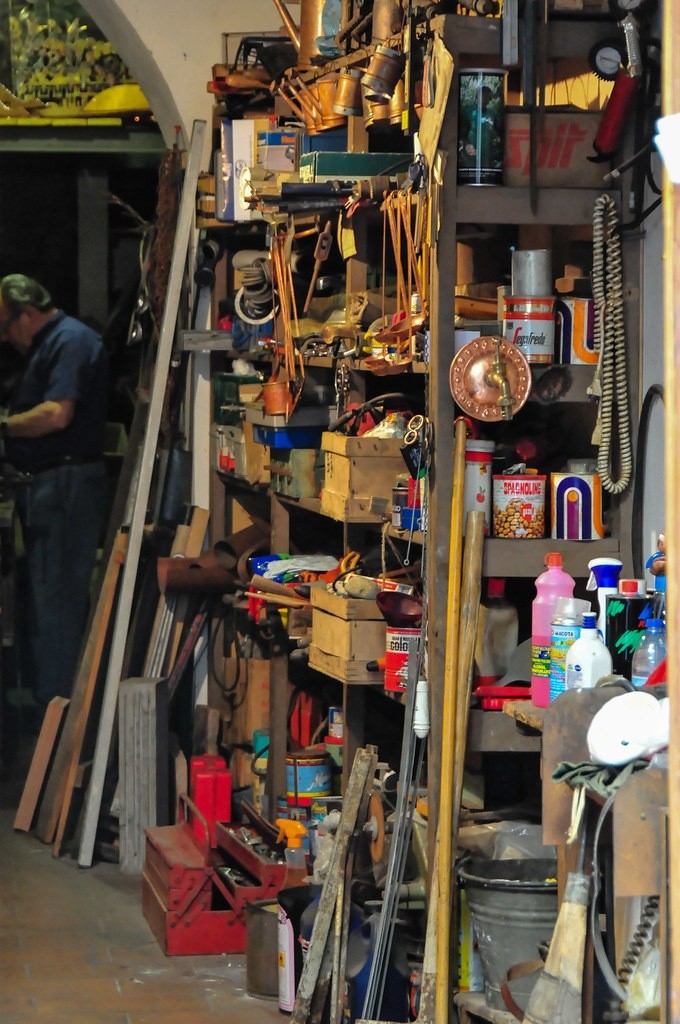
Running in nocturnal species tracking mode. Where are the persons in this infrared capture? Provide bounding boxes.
[0,274,113,719]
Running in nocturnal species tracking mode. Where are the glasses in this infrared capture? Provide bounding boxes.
[1,316,16,332]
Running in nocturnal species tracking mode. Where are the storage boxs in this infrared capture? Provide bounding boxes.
[207,152,415,685]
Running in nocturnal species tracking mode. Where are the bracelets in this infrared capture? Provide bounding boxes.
[0,417,7,438]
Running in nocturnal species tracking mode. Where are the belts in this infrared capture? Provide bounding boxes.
[22,451,99,473]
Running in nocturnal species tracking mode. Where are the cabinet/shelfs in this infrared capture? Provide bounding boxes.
[192,14,641,751]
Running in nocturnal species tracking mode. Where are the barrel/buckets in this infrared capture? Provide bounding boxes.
[241,897,279,1002]
[456,857,559,1015]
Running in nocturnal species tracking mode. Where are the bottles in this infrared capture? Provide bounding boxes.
[621,580,638,599]
[632,618,666,687]
[531,552,576,708]
[549,595,590,703]
[565,612,613,689]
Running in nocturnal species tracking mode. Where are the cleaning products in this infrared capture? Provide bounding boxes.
[530,549,668,709]
